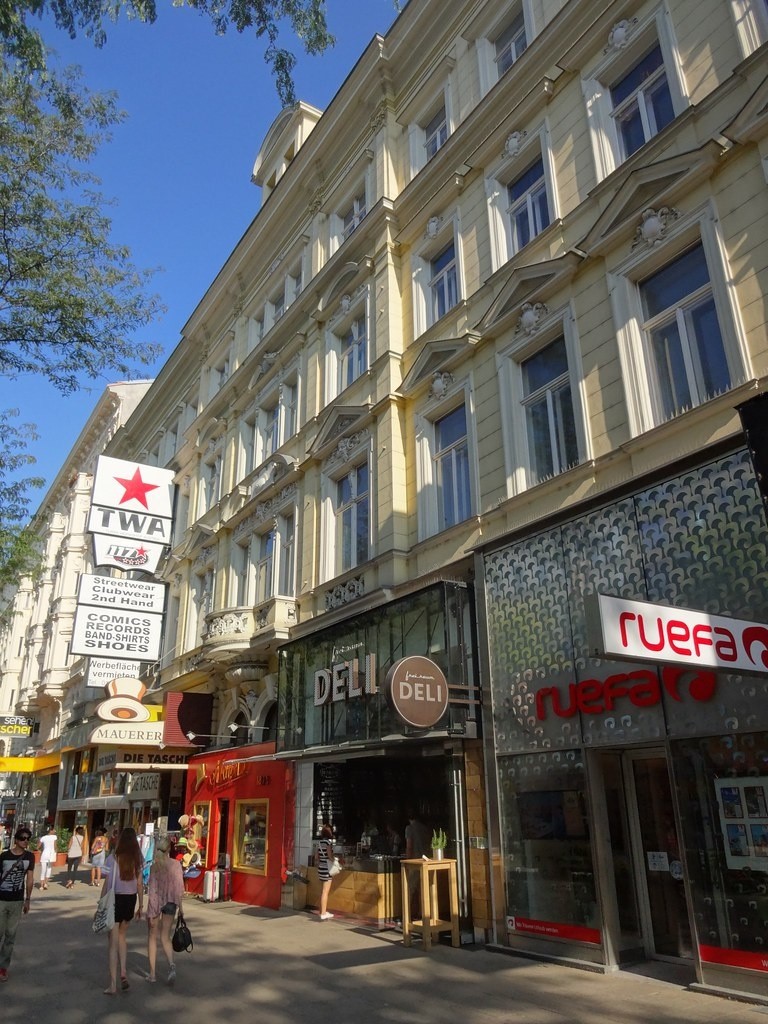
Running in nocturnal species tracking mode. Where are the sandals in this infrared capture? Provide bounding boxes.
[168,964,176,985]
[103,987,117,994]
[145,976,157,983]
[120,977,129,990]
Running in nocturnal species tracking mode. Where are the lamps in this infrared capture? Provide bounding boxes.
[186,731,238,743]
[227,724,276,732]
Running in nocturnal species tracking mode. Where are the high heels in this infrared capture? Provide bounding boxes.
[89,882,100,887]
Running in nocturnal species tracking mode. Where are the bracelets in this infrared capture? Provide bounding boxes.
[139,906,143,908]
[26,898,30,900]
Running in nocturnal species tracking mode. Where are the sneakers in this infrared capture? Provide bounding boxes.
[0,969,8,981]
[319,912,334,919]
[39,884,48,891]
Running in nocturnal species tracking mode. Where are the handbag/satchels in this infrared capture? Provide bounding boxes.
[326,858,341,876]
[90,842,102,854]
[172,927,193,952]
[93,889,115,935]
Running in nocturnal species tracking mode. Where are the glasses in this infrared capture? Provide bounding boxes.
[18,836,30,841]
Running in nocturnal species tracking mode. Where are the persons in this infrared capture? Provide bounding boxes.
[397,807,430,928]
[385,822,402,852]
[100,828,144,994]
[91,827,108,887]
[145,838,185,986]
[0,828,35,981]
[65,827,85,889]
[37,825,58,891]
[314,825,339,919]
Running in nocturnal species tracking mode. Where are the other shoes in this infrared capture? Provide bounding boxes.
[66,881,75,889]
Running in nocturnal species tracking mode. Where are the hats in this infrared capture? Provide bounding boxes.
[175,814,204,878]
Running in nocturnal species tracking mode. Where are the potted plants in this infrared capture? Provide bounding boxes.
[431,828,447,860]
[29,827,73,867]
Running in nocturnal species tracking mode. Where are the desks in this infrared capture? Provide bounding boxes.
[400,859,460,951]
